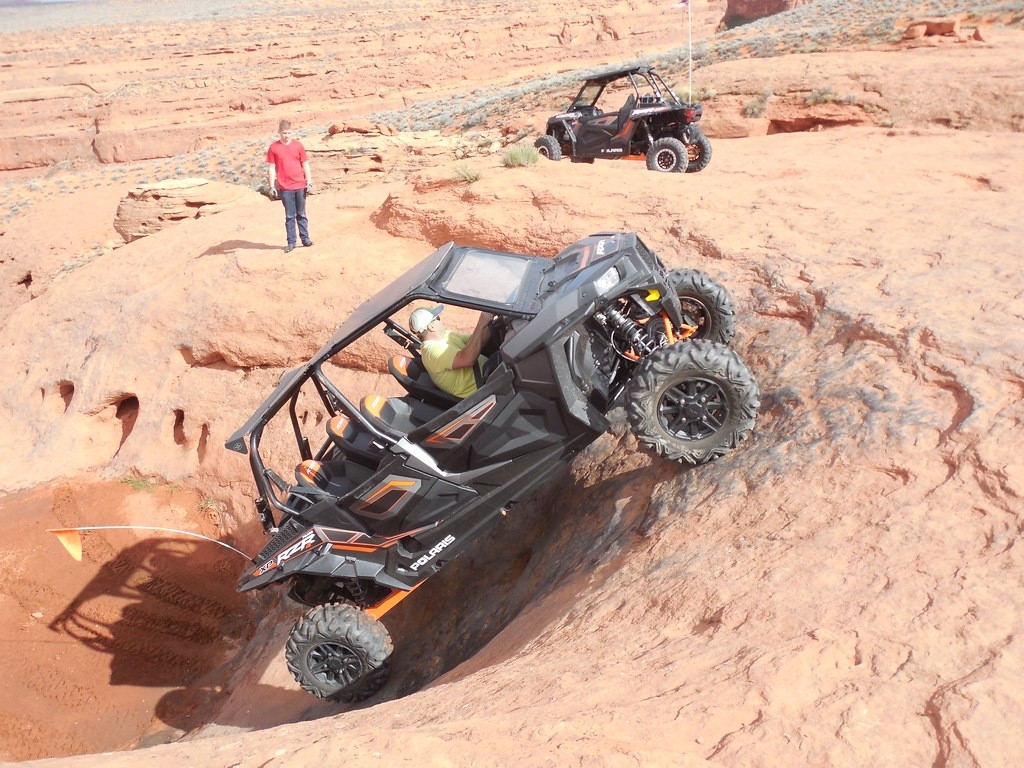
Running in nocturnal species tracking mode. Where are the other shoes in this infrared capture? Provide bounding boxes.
[303,241,315,246]
[286,244,295,252]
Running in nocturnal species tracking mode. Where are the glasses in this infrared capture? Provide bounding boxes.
[425,315,441,330]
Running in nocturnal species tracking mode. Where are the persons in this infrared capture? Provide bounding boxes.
[266,120,314,253]
[409,303,498,400]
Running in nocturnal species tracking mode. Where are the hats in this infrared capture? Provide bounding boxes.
[408,304,445,333]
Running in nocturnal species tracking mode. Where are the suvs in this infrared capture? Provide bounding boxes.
[222,230,762,707]
[532,62,712,176]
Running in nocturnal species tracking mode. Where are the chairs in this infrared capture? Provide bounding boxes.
[295,460,375,497]
[326,414,385,469]
[359,394,443,438]
[388,355,462,408]
